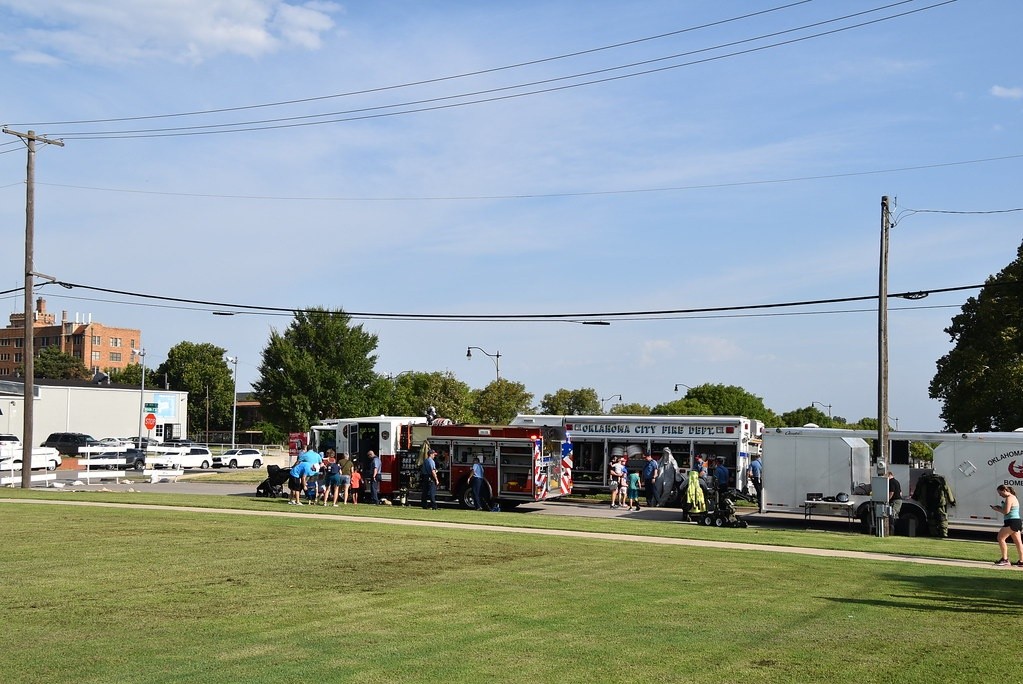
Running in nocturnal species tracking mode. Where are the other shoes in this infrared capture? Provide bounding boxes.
[432,508,441,510]
[296,503,304,506]
[636,507,640,511]
[644,504,652,507]
[654,504,663,507]
[323,503,327,506]
[332,503,338,506]
[288,500,296,505]
[477,507,482,510]
[627,507,633,511]
[610,504,619,509]
[618,504,628,508]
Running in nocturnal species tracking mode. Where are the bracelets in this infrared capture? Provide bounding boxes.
[651,477,656,479]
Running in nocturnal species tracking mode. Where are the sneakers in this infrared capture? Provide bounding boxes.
[1012,560,1023,567]
[996,558,1009,565]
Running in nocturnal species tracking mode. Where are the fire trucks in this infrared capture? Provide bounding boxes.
[509,410,765,502]
[306,417,574,512]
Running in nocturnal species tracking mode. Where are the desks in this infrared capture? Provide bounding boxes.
[804,500,855,525]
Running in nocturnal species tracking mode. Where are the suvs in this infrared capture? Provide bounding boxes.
[211,449,264,469]
[44,432,99,457]
[0,447,62,471]
[152,448,213,470]
[0,434,23,454]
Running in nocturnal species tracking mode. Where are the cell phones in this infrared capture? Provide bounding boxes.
[990,505,992,507]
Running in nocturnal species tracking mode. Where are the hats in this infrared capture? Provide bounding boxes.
[313,463,320,472]
[643,452,651,458]
[621,459,627,462]
[428,450,437,455]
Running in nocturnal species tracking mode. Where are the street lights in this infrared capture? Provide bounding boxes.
[601,395,622,413]
[132,348,148,449]
[226,356,238,449]
[466,346,502,381]
[812,402,832,417]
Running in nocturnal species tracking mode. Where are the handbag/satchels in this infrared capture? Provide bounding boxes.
[855,483,872,496]
[609,481,618,490]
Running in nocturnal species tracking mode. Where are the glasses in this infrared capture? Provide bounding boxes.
[473,460,475,462]
[368,454,371,456]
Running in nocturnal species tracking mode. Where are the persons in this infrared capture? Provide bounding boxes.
[713,458,729,510]
[642,452,661,507]
[887,472,902,535]
[421,450,441,510]
[288,445,382,507]
[992,484,1023,567]
[609,455,628,508]
[467,458,484,511]
[626,469,641,511]
[749,454,763,513]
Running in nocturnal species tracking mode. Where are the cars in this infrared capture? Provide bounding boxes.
[129,437,157,447]
[100,437,136,449]
[164,438,194,446]
[86,448,145,471]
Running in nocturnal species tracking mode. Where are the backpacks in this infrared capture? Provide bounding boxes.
[325,463,339,474]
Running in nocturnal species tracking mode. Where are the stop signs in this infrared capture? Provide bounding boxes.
[145,414,156,430]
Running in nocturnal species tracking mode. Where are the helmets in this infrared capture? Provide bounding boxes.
[924,468,937,477]
[699,473,708,481]
